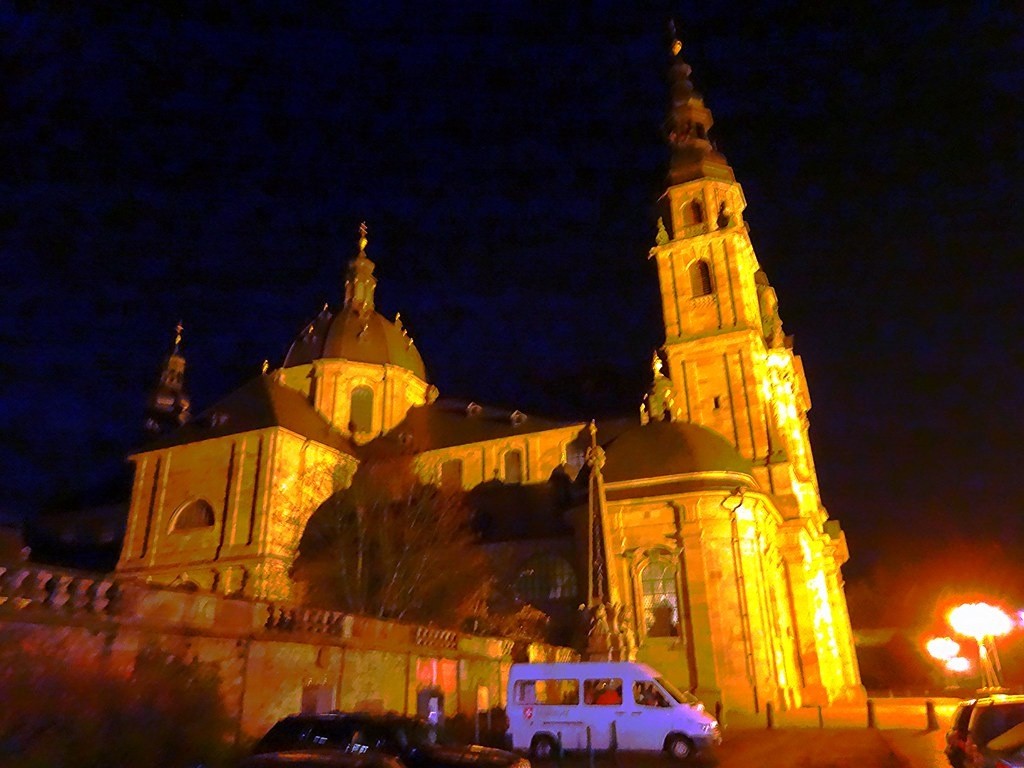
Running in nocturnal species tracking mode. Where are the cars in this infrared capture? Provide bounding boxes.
[944,694,1024,768]
[241,711,533,768]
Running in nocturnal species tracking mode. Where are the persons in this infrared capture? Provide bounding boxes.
[593,684,622,705]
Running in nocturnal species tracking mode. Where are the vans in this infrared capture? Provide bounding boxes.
[503,660,722,763]
